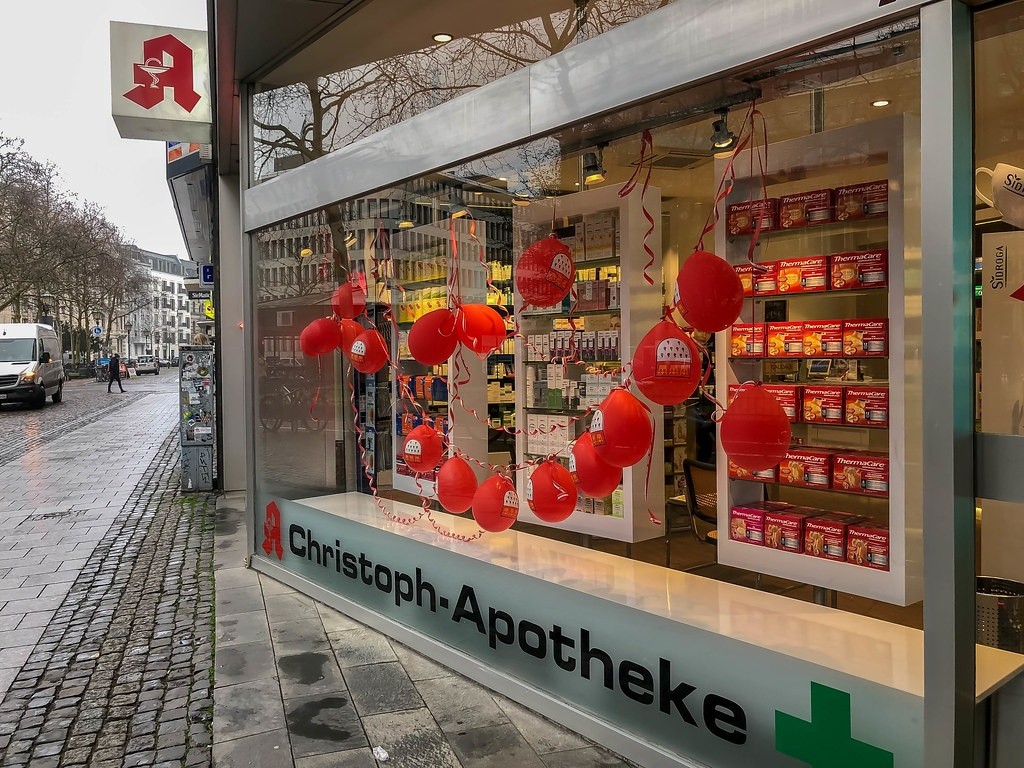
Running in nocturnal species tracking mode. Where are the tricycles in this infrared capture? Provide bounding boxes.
[94,358,111,382]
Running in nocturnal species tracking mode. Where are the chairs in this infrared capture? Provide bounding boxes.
[665,457,808,594]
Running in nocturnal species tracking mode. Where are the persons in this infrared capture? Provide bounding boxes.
[107,353,127,393]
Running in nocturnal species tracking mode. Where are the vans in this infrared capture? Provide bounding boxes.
[0,323,64,410]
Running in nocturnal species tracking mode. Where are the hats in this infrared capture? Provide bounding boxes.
[115,353,120,357]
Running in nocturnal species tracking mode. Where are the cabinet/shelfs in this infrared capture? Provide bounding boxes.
[714,112,924,607]
[485,258,516,453]
[512,182,667,542]
[389,218,489,503]
[356,300,392,490]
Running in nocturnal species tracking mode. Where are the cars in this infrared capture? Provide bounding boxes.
[125,357,179,369]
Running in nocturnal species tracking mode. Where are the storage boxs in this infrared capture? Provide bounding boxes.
[729,447,889,497]
[731,318,889,358]
[727,384,888,428]
[730,501,889,572]
[522,214,624,519]
[397,245,516,483]
[731,248,888,297]
[727,179,889,236]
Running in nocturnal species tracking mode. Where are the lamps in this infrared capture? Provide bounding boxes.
[450,205,468,220]
[399,204,414,229]
[300,247,313,258]
[512,191,531,207]
[710,108,740,159]
[583,142,609,185]
[343,233,358,248]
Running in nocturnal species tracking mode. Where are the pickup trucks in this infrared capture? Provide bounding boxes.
[135,355,160,376]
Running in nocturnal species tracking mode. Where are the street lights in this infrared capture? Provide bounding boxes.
[92,308,104,358]
[166,333,172,361]
[154,332,160,357]
[40,289,56,324]
[124,322,133,367]
[143,328,150,355]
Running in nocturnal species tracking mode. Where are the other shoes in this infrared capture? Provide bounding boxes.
[108,391,114,393]
[120,389,127,393]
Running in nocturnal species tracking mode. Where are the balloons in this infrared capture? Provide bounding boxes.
[295,214,802,534]
